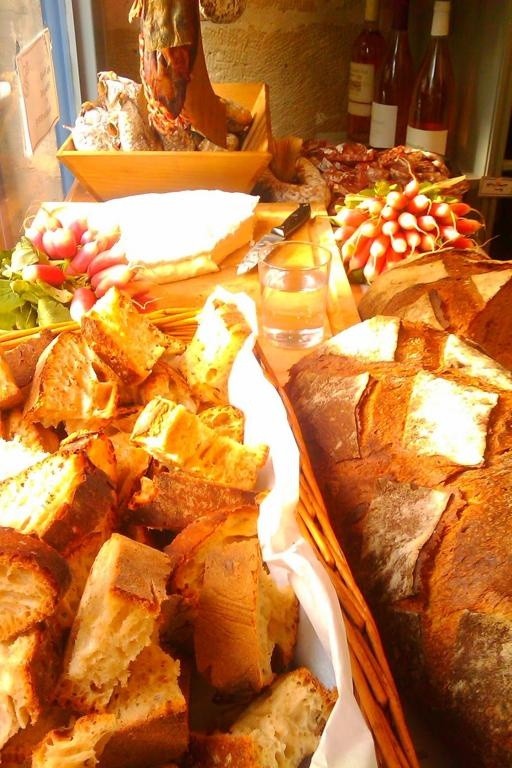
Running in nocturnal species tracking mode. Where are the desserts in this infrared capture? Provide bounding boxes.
[0,288,347,768]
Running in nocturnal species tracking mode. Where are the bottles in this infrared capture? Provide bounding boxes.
[344,0,387,142]
[370,1,413,151]
[405,0,456,161]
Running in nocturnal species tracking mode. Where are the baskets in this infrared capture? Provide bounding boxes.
[0,300,419,768]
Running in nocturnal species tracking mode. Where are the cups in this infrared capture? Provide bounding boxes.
[255,241,333,350]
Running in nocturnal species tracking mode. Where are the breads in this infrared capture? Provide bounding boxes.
[357,244,512,367]
[71,69,251,152]
[288,314,511,767]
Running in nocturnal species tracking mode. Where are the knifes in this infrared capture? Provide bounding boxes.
[237,200,313,273]
